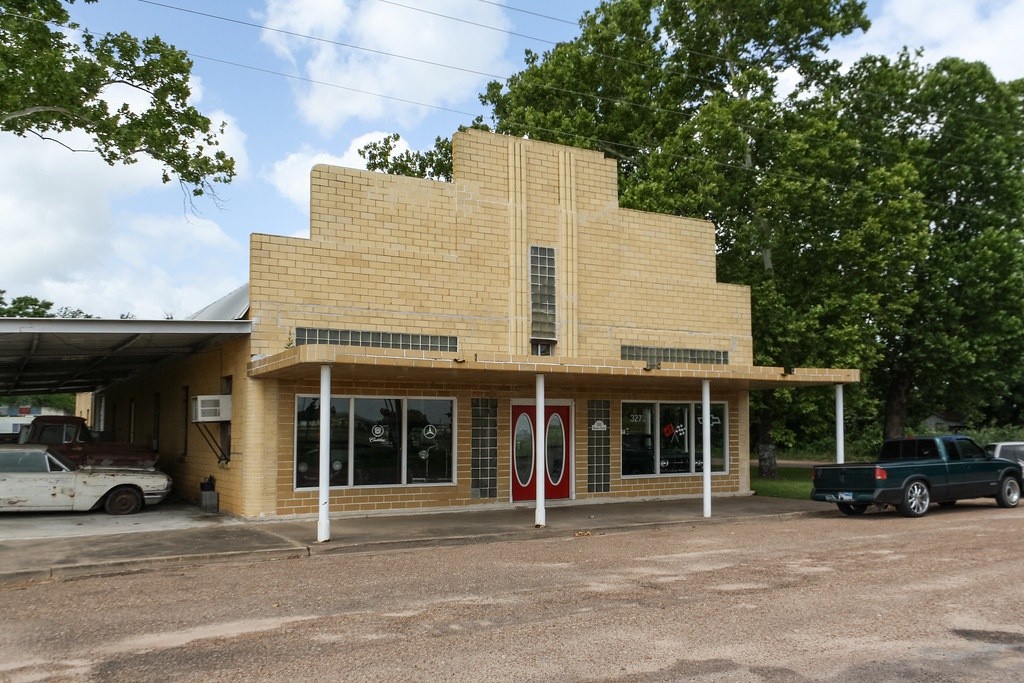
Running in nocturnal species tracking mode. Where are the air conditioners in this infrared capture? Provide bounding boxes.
[191,394,232,423]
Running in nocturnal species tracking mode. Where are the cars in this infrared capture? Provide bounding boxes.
[298,451,363,487]
[984,441,1024,479]
[0,415,174,516]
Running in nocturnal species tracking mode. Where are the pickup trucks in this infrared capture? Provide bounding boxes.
[809,435,1024,517]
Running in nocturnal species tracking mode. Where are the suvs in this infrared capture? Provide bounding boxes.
[622,432,704,472]
